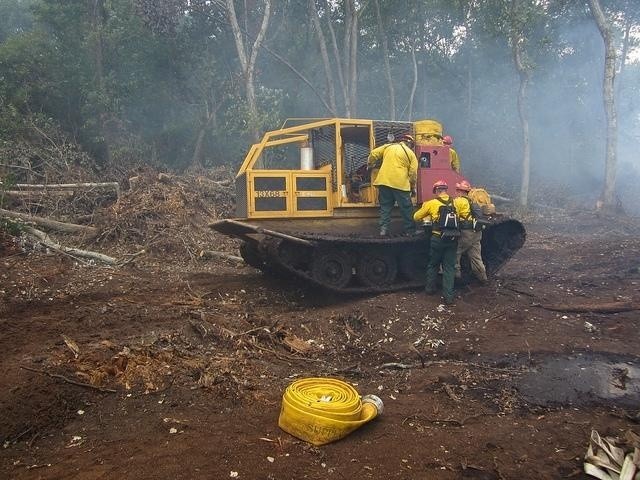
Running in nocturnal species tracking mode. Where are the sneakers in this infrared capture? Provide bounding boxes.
[379,227,426,238]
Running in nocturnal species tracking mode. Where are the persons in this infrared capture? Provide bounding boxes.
[414,178,488,310]
[443,134,460,176]
[366,133,419,235]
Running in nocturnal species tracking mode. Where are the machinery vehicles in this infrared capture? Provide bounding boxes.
[207,117,527,295]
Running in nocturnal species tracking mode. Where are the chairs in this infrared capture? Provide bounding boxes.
[355,157,379,185]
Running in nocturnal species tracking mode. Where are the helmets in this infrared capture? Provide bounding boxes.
[442,136,453,144]
[432,181,449,194]
[399,134,415,144]
[456,181,471,192]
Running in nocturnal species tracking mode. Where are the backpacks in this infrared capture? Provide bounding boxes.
[436,197,458,228]
[464,188,497,231]
